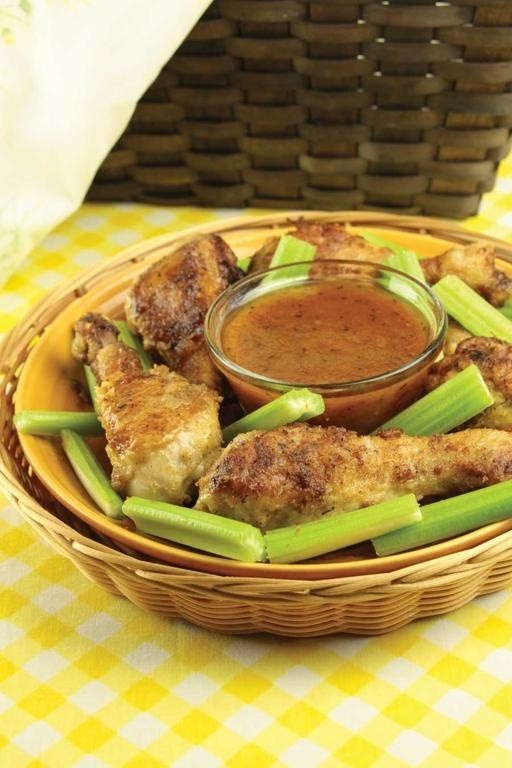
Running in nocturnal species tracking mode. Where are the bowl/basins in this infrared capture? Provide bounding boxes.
[200,257,449,434]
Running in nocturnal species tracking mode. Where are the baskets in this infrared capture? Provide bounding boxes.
[0,206,512,641]
[81,0,511,221]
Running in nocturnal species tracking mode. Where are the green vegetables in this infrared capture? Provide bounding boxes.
[366,364,494,435]
[83,363,102,414]
[265,235,318,283]
[378,252,430,314]
[371,481,510,557]
[266,493,423,564]
[430,274,511,343]
[222,389,326,448]
[59,429,123,517]
[114,320,152,372]
[359,232,401,260]
[122,496,267,563]
[16,410,106,437]
[237,258,250,272]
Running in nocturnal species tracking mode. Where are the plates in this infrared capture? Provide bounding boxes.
[13,228,511,582]
